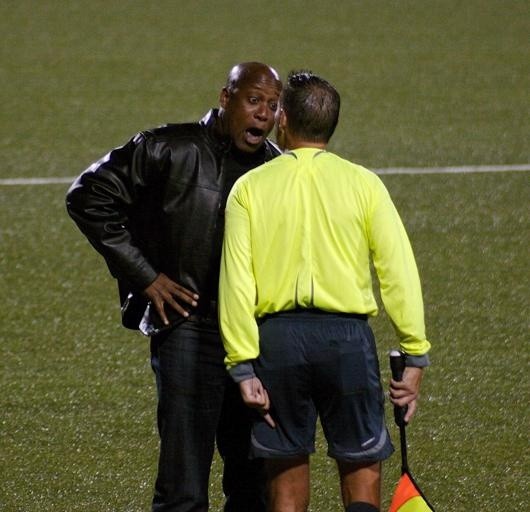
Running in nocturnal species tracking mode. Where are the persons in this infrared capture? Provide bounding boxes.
[216,70,431,512]
[65,61,282,510]
